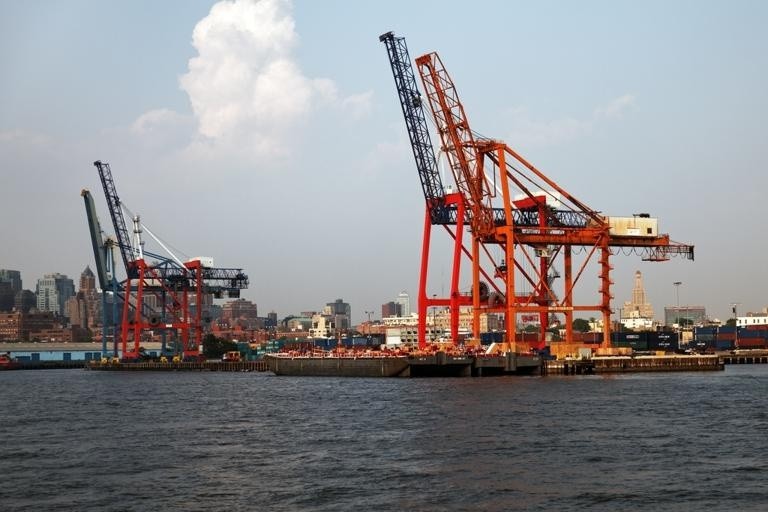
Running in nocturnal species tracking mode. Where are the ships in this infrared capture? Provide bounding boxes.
[264,350,473,379]
